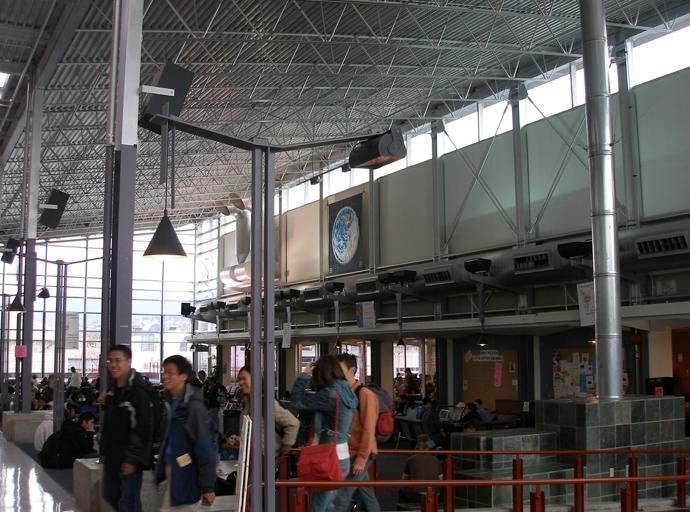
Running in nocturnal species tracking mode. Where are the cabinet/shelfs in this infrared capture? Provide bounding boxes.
[10,397,14,413]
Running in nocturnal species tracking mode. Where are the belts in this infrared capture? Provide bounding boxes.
[93,433,103,464]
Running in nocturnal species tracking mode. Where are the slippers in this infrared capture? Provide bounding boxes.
[119,374,163,438]
[38,423,86,470]
[353,383,394,444]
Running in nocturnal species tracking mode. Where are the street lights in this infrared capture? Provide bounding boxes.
[295,442,344,494]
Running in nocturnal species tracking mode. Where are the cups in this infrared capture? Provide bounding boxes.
[105,358,128,364]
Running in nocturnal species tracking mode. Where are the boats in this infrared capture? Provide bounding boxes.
[38,188,70,228]
[0,238,20,264]
[139,60,194,137]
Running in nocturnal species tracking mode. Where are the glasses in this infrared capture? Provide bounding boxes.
[140,64,193,258]
[1,237,27,313]
[36,182,69,299]
[346,131,408,172]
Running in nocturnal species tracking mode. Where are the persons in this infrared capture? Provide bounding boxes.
[335,353,380,510]
[238,366,300,510]
[8,367,100,468]
[98,355,216,511]
[100,345,155,510]
[393,369,487,451]
[198,370,241,496]
[289,354,357,510]
[400,434,446,502]
